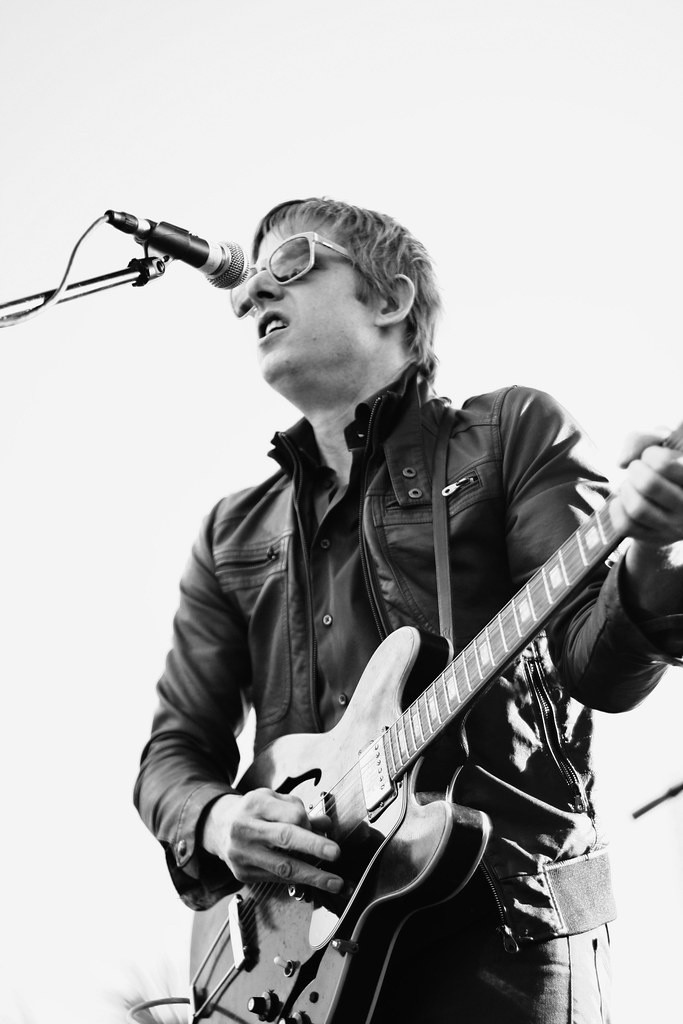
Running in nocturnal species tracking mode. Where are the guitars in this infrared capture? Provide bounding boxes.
[185,418,683,1024]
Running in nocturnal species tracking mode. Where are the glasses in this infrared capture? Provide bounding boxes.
[229,230,392,319]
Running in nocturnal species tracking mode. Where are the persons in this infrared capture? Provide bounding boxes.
[131,194,683,1024]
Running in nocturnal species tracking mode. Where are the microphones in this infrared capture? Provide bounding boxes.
[105,210,249,290]
